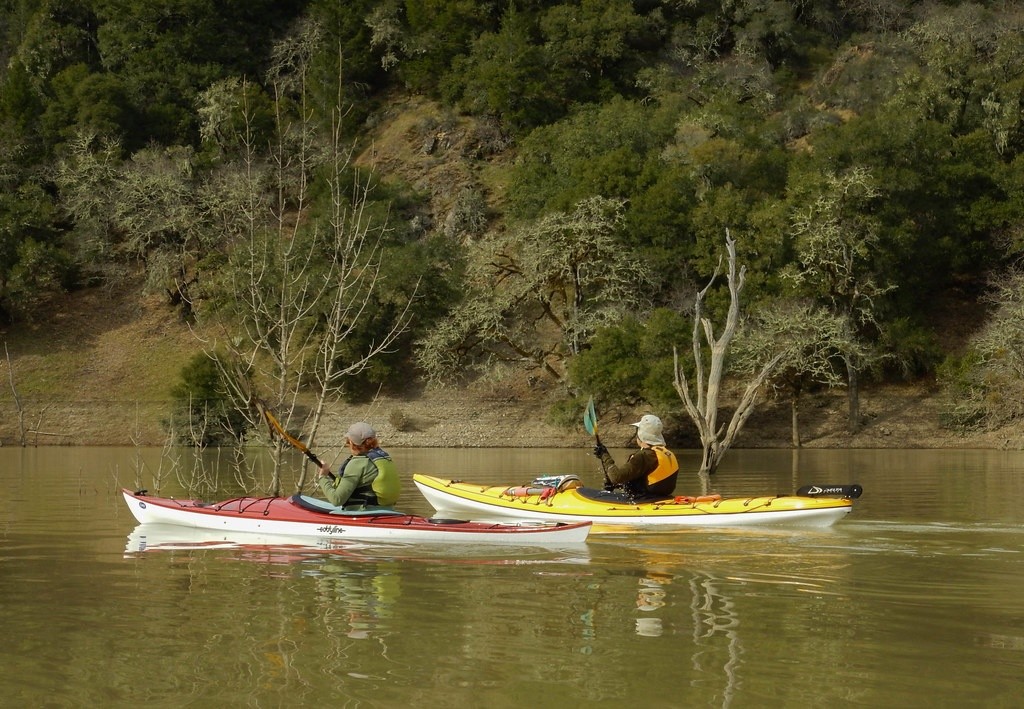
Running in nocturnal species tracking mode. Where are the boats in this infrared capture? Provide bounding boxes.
[118,486,593,544]
[411,473,865,526]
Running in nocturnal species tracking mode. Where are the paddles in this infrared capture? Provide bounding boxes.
[583,395,616,493]
[265,411,337,481]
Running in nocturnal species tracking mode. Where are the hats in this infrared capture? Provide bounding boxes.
[345,422,376,446]
[629,415,666,446]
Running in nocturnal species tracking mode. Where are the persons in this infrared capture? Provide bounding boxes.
[593,414,679,502]
[319,422,396,511]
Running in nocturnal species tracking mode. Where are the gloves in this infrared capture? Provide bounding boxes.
[594,444,607,458]
